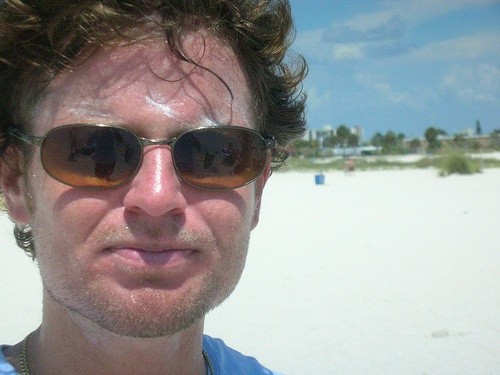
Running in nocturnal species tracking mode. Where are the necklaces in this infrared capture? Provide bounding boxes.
[16,328,217,375]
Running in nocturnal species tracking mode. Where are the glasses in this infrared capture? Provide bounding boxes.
[8,126,277,191]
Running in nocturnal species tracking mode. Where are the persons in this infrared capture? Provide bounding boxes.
[0,0,312,375]
